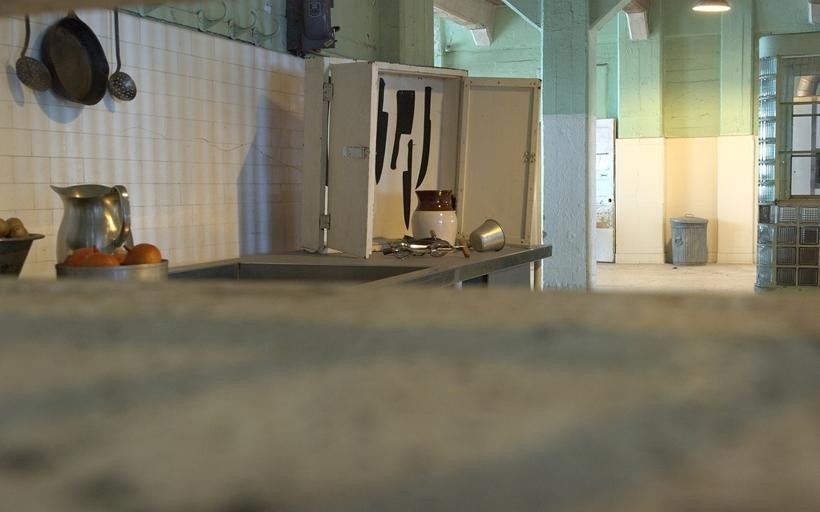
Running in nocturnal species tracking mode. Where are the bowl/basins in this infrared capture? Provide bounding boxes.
[1,231,45,277]
[469,218,506,252]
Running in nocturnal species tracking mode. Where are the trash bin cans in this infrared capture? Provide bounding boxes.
[670,213,708,266]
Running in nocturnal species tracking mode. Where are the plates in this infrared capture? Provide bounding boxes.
[54,258,169,281]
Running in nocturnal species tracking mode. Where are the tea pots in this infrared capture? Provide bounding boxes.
[49,183,135,263]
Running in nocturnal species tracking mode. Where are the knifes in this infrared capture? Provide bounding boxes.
[389,89,416,170]
[375,77,389,185]
[415,86,432,190]
[402,139,413,231]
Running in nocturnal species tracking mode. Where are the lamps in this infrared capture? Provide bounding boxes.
[692,0,734,16]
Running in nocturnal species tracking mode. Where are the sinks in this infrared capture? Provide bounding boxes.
[167,261,432,289]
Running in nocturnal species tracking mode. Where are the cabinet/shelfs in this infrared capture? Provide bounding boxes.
[292,55,471,258]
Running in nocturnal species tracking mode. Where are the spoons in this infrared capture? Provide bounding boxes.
[15,15,52,92]
[109,10,136,101]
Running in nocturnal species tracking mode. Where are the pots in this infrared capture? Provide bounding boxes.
[40,12,109,105]
